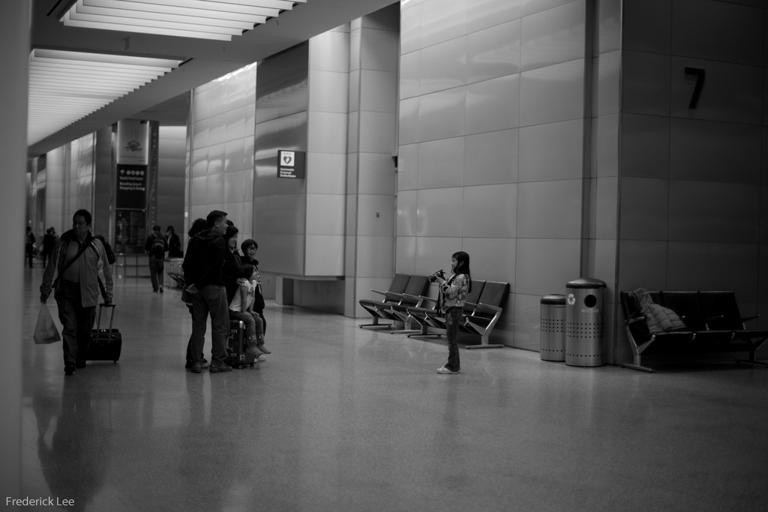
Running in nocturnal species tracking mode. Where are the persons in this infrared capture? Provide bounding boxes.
[25,226,35,266]
[181,208,271,372]
[163,225,180,259]
[145,225,167,294]
[437,251,473,373]
[38,209,114,373]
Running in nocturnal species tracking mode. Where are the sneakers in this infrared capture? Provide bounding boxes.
[257,343,271,355]
[437,366,456,374]
[247,344,263,358]
[191,358,232,373]
[66,367,79,376]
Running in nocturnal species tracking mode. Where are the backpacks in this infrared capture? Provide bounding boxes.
[151,233,165,259]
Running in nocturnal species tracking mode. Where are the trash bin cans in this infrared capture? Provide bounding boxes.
[540,294,566,362]
[566,278,606,366]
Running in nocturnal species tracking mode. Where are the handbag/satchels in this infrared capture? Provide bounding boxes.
[51,275,80,318]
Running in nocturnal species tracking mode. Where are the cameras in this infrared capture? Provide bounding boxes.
[428,270,444,282]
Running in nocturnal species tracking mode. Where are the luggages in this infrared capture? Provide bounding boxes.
[226,319,256,370]
[87,303,122,363]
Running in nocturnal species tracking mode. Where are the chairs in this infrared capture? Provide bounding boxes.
[358,274,510,351]
[619,287,767,375]
